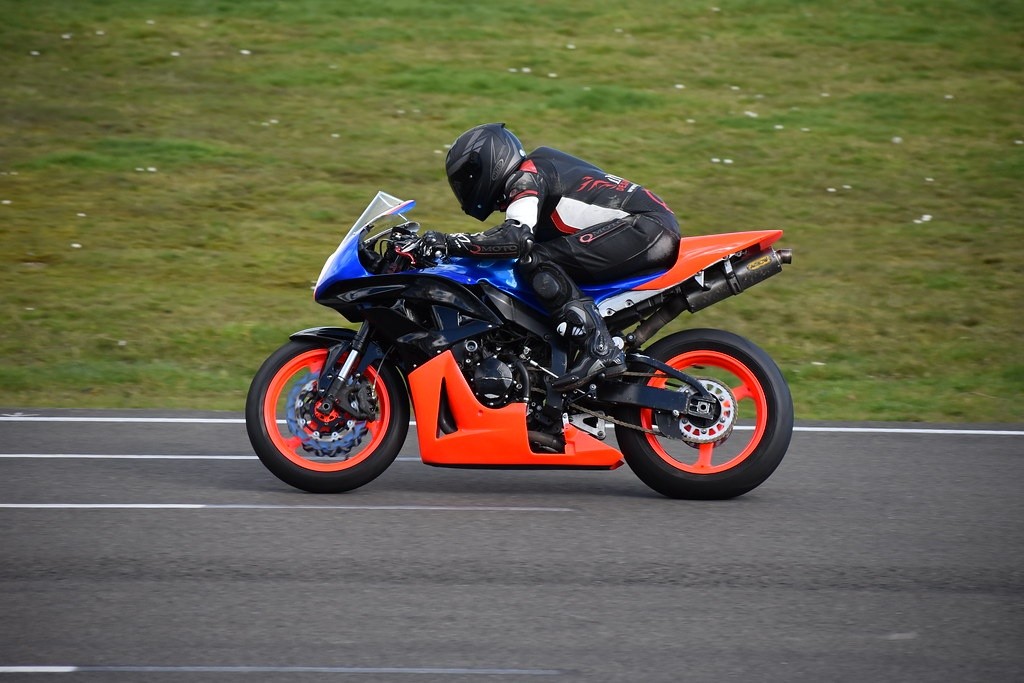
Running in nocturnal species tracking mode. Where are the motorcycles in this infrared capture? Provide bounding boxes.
[245,190,795,502]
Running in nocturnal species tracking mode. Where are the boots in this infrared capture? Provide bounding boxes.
[550,297,627,391]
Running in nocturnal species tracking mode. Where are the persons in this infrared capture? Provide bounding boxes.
[400,122,681,392]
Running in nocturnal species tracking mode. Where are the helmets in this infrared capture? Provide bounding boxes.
[446,122,527,222]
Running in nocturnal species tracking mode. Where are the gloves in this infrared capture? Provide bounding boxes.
[420,229,450,260]
